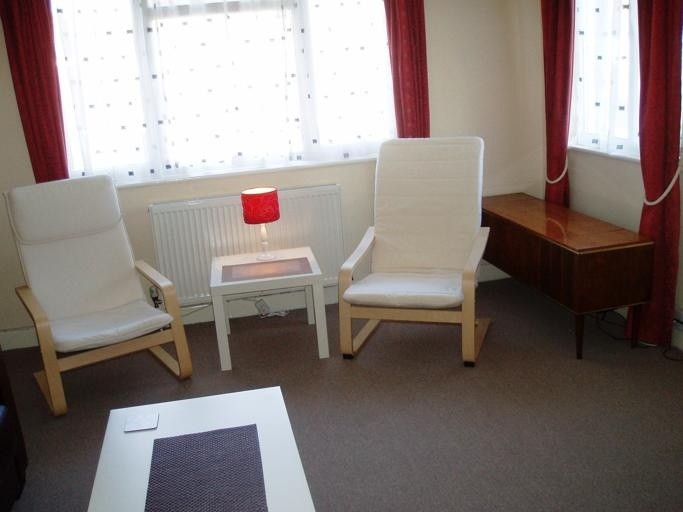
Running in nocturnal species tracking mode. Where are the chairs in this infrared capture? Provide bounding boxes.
[0,172,192,417]
[336,135,494,367]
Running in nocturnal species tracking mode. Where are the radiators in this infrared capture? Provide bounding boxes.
[144,183,354,309]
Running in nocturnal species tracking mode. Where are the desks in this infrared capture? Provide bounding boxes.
[208,246,333,370]
[481,191,655,360]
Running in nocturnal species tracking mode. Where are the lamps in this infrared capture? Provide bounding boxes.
[239,185,280,261]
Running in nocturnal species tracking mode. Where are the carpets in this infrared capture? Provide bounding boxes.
[87,384,318,512]
